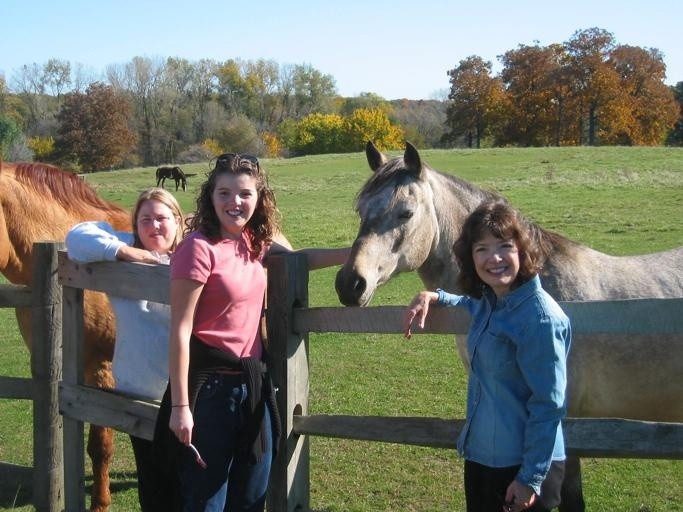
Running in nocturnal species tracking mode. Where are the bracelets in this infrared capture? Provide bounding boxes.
[170,404,189,408]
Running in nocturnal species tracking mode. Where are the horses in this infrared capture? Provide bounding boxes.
[0,159,296,512]
[333,139,683,512]
[156,167,187,193]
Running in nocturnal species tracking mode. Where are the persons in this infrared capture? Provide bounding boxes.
[150,153,282,512]
[404,201,572,512]
[64,187,185,512]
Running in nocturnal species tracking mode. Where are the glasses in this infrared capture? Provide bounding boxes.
[216,154,259,173]
